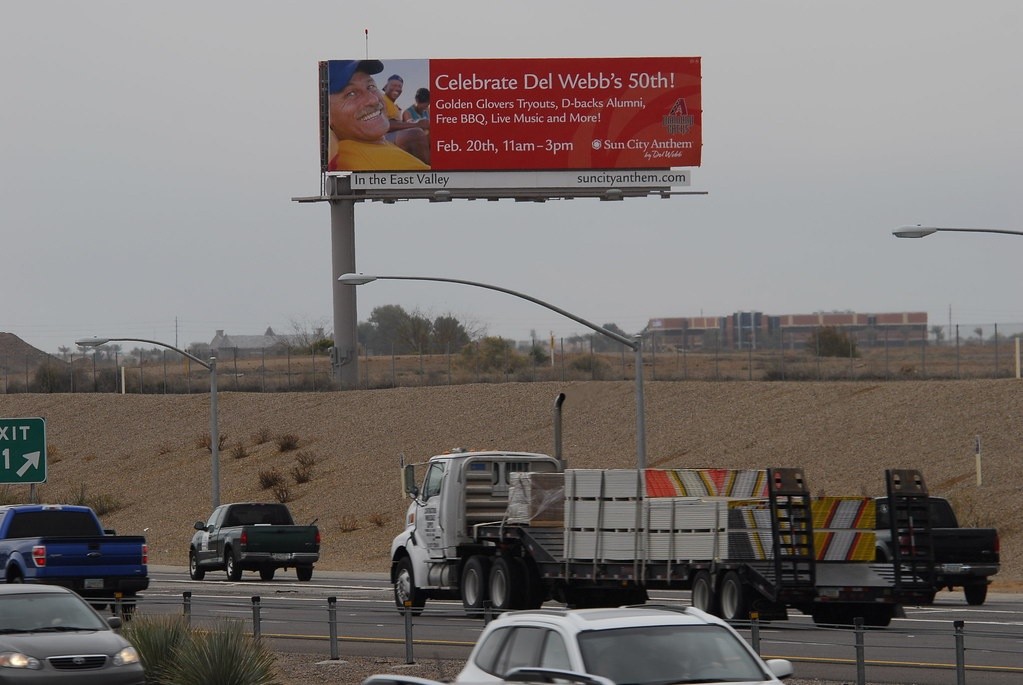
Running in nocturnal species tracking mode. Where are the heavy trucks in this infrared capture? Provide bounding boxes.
[389,390,946,631]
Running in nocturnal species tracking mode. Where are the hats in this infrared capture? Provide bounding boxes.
[330,60,384,94]
[381,74,403,92]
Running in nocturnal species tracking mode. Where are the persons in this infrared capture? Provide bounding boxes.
[329,60,429,170]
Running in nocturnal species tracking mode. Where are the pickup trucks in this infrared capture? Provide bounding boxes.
[876,496,1003,607]
[188,501,322,582]
[0,501,150,621]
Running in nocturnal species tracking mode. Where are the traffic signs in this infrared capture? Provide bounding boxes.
[0,417,49,485]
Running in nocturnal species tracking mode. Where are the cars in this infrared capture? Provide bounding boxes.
[0,583,146,685]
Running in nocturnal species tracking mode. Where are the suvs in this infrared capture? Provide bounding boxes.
[453,601,794,685]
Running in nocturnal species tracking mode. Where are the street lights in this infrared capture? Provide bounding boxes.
[338,272,648,470]
[74,336,221,509]
